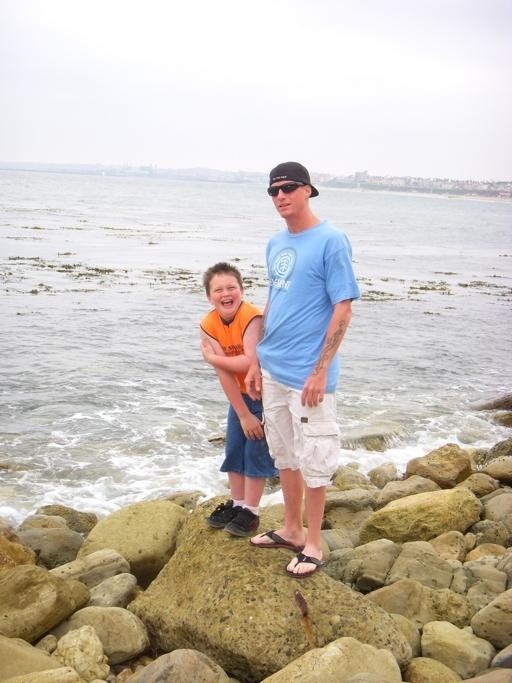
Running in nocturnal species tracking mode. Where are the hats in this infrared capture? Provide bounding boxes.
[270,162,319,198]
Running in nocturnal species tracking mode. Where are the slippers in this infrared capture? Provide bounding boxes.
[249,530,305,552]
[286,550,330,578]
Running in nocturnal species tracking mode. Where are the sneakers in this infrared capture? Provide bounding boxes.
[207,500,242,527]
[223,508,260,537]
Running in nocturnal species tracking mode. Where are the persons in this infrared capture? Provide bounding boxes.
[243,162,360,579]
[197,263,265,538]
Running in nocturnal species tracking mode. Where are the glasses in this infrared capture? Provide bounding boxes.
[267,182,304,196]
